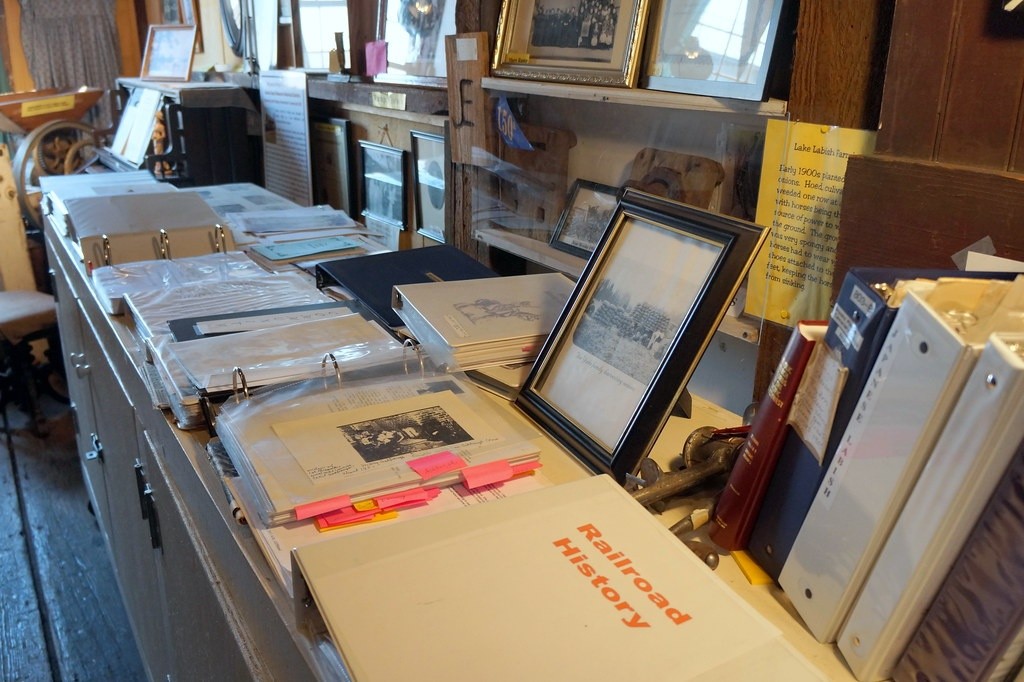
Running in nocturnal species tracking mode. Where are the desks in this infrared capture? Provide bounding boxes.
[39,185,901,682]
[116,76,259,185]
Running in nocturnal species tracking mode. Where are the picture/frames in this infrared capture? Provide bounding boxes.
[140,0,204,83]
[488,0,651,91]
[408,130,445,243]
[549,178,622,259]
[512,188,772,493]
[641,0,792,102]
[356,139,408,231]
